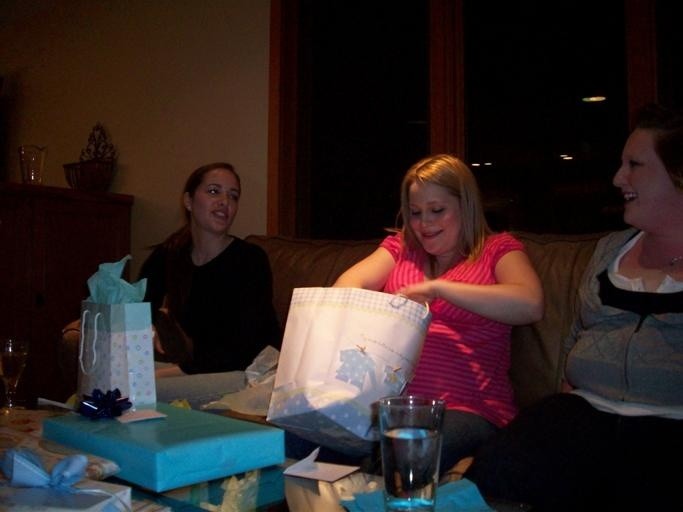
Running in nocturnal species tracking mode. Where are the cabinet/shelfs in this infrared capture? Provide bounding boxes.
[0,180,134,407]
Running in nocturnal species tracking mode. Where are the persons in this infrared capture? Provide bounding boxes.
[62,162,280,411]
[329,154,544,485]
[439,99,683,512]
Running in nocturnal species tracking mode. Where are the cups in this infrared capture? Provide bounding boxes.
[1,335,29,417]
[17,143,48,185]
[377,398,445,512]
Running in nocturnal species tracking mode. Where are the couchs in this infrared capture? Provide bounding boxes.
[60,231,598,475]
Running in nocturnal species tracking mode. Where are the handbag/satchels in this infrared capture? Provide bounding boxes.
[266,280,431,463]
[78,301,156,406]
